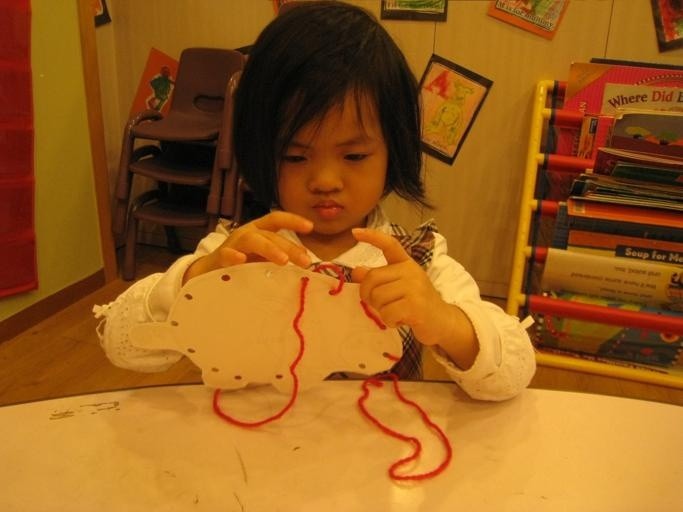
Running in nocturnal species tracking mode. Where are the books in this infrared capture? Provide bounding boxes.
[557,108,683,267]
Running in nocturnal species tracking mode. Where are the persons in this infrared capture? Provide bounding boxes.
[94,2,537,401]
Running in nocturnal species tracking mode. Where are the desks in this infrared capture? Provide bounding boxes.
[0,387,683,512]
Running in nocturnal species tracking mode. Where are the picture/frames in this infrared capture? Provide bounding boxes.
[486,0,571,41]
[380,0,448,24]
[417,53,494,168]
[650,0,683,54]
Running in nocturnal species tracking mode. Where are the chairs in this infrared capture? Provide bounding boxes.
[111,46,246,282]
